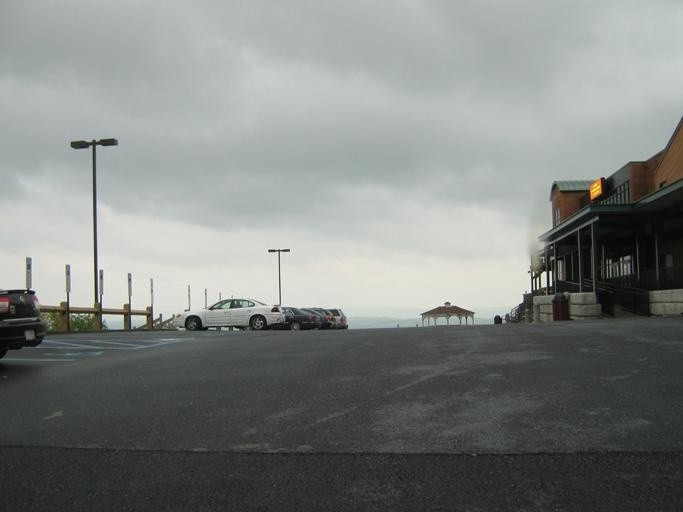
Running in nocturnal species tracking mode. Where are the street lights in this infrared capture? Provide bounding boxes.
[72,139,118,303]
[268,249,290,306]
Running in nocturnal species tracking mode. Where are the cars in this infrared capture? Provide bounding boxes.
[173,298,285,331]
[0,290,49,359]
[282,307,348,330]
